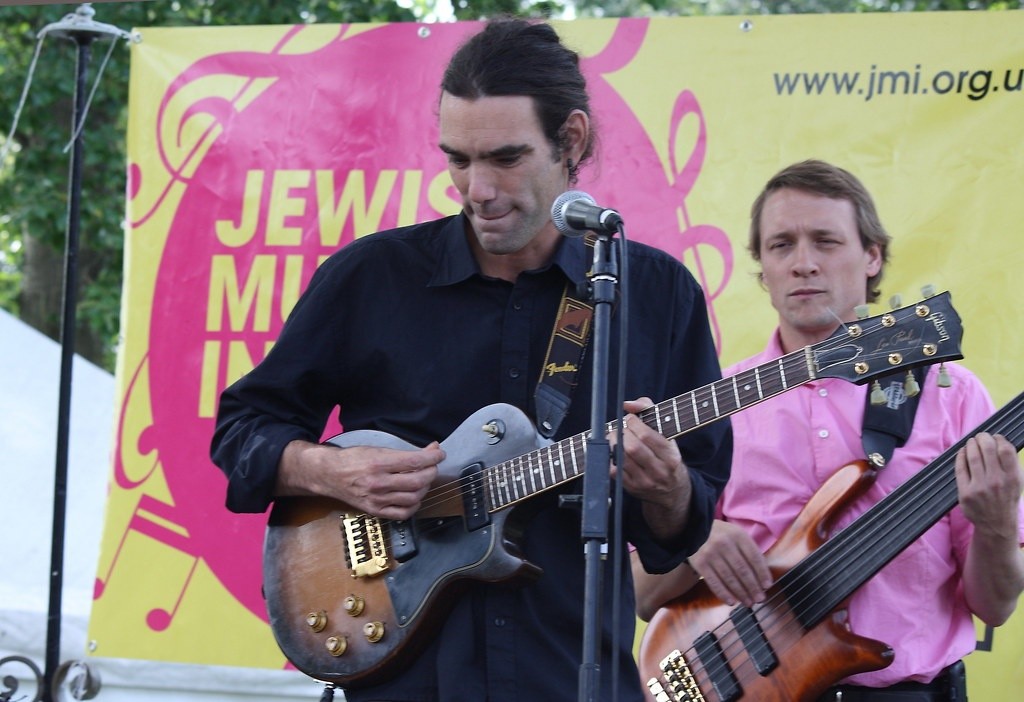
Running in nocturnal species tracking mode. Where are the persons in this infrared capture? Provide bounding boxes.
[208,21,735,702]
[622,157,1024,702]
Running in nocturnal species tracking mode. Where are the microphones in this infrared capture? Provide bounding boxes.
[552,190,624,238]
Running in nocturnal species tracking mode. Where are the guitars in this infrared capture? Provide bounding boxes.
[638,391,1024,702]
[260,285,966,688]
